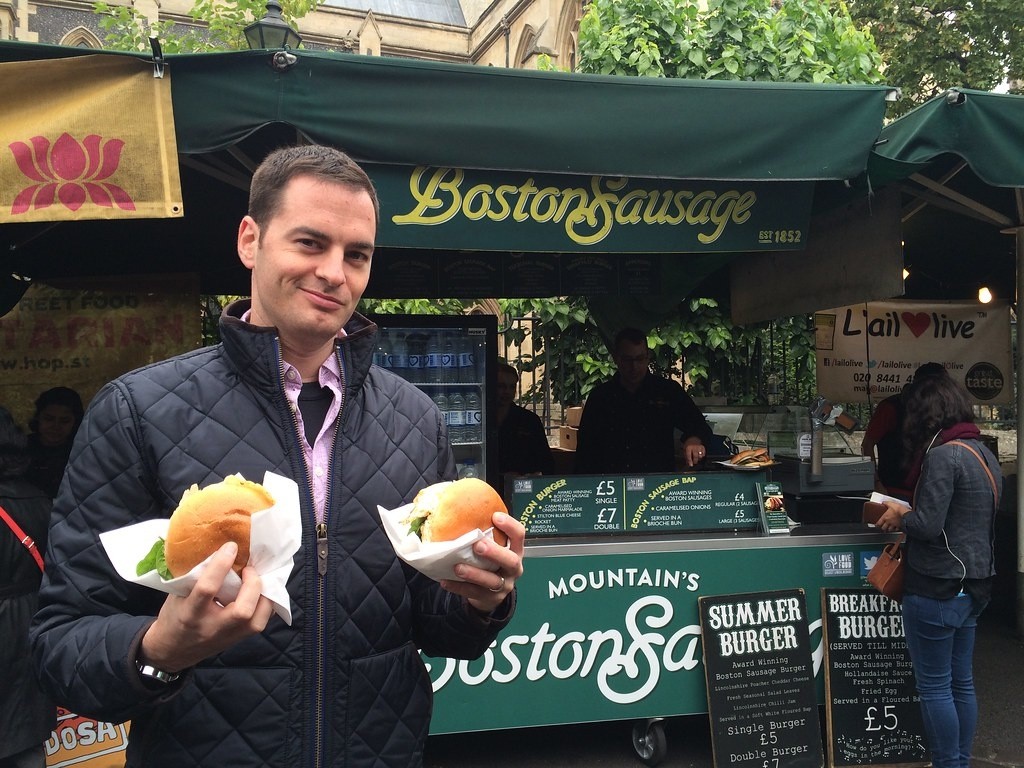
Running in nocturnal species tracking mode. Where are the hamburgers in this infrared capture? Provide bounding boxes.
[135,473,277,578]
[401,477,512,547]
[731,450,760,466]
[755,447,774,466]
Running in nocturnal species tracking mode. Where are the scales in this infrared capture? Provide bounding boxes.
[772,399,876,499]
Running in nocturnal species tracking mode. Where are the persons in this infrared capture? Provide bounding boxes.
[31,141,527,768]
[496,361,556,474]
[873,373,1003,768]
[0,407,61,768]
[16,387,83,497]
[860,360,949,507]
[577,327,718,473]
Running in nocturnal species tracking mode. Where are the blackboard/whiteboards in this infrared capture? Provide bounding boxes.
[819,585,934,768]
[692,586,826,768]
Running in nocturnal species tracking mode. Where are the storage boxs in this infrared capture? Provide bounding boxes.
[558,422,580,451]
[565,405,584,428]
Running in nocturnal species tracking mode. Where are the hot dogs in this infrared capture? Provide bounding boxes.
[764,498,774,511]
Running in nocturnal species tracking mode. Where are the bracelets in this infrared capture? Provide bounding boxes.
[873,478,881,485]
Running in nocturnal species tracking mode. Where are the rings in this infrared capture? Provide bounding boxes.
[489,572,506,593]
[698,452,703,455]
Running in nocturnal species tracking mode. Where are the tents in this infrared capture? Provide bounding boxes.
[880,84,1023,242]
[0,39,890,322]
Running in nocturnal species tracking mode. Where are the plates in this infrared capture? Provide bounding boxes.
[714,459,782,469]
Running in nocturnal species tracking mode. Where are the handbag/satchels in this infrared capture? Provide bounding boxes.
[866,532,905,603]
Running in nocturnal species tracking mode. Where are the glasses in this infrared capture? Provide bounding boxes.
[614,352,648,364]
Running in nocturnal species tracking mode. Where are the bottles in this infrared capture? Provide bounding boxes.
[373,327,483,481]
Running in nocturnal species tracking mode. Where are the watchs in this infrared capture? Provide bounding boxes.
[134,658,183,685]
